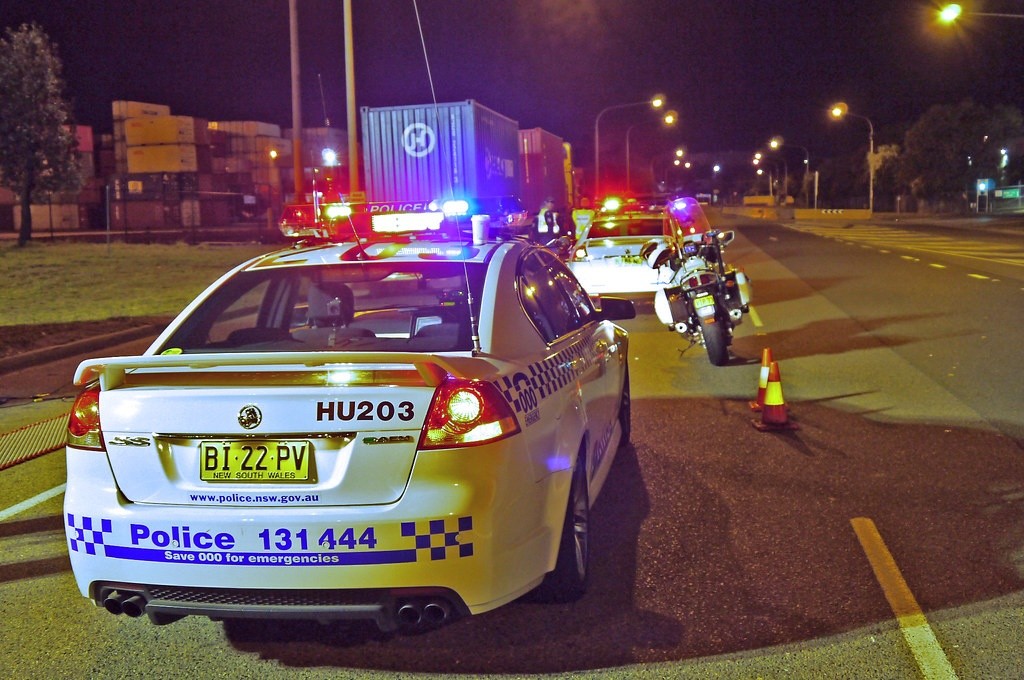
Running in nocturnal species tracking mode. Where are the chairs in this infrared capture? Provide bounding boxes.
[290,281,376,343]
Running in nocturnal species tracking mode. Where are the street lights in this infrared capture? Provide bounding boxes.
[595,100,662,195]
[833,108,874,212]
[769,140,809,207]
[755,153,788,195]
[627,115,674,189]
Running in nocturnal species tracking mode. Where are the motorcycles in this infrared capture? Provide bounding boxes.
[655,197,753,365]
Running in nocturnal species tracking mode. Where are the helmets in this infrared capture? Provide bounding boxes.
[642,235,673,268]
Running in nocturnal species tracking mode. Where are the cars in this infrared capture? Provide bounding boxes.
[63,204,632,634]
[570,202,683,296]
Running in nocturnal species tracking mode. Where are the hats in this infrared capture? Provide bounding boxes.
[545,196,556,202]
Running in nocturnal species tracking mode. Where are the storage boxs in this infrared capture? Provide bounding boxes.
[10,99,349,236]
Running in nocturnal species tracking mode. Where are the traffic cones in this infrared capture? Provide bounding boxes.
[749,347,790,411]
[751,360,800,430]
